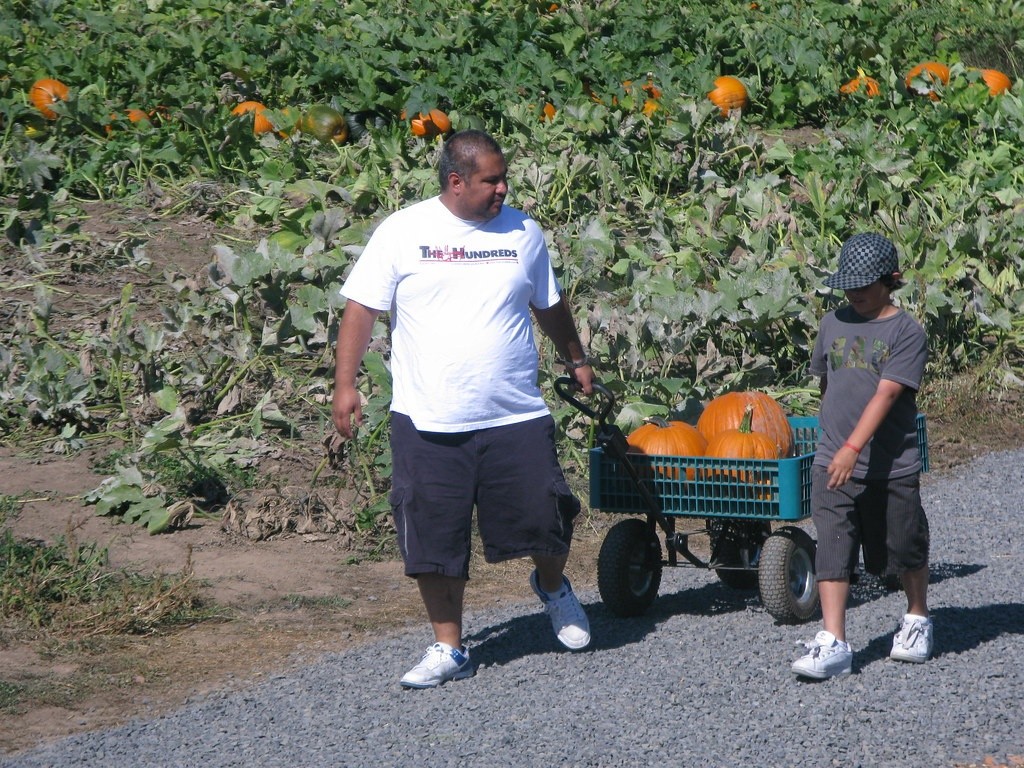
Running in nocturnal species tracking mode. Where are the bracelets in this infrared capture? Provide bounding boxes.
[565,355,588,369]
[844,441,861,454]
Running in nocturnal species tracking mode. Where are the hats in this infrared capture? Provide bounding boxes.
[821,232,899,290]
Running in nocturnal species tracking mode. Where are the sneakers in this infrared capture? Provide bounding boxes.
[890,613,935,662]
[791,630,853,678]
[529,568,590,650]
[400,642,474,688]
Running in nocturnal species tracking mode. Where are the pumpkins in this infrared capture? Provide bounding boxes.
[621,382,792,501]
[19,76,748,145]
[839,61,1012,103]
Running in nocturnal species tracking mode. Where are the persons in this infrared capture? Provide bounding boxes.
[791,232,935,680]
[332,128,596,690]
